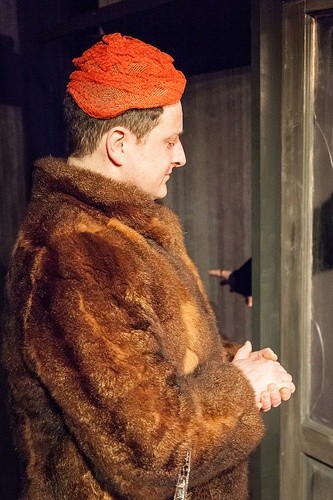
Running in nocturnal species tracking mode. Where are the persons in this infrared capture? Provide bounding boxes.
[206,199,333,305]
[3,33,296,500]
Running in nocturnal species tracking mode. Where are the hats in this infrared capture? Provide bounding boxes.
[66,32,187,120]
